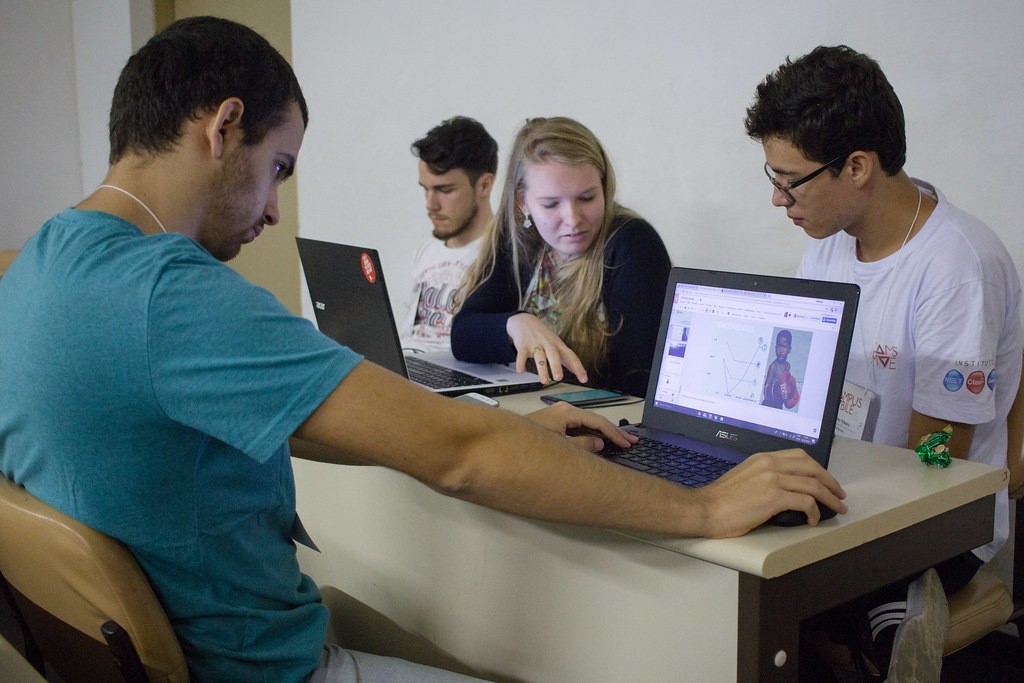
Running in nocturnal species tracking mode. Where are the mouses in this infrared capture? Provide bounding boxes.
[766,497,837,528]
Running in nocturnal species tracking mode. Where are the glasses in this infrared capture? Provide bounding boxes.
[761,143,867,203]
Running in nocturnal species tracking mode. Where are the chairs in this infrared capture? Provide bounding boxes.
[0,473,187,683]
[863,355,1024,676]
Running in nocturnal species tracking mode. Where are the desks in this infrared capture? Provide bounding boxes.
[292,344,1010,683]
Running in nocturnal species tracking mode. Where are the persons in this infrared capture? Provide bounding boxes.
[450,113,672,399]
[762,330,799,410]
[390,117,499,354]
[749,42,1023,683]
[0,16,850,683]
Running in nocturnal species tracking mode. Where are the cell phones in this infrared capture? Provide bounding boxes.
[539,388,631,406]
[455,392,499,408]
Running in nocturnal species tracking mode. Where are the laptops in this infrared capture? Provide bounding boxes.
[295,237,545,397]
[583,266,861,489]
